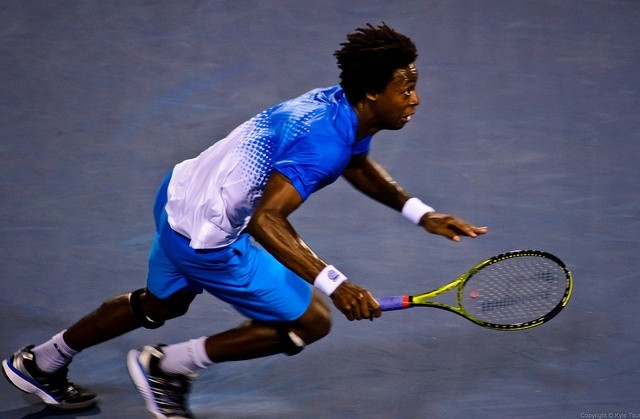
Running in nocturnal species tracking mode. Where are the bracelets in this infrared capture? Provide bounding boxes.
[402,197,435,226]
[313,264,348,296]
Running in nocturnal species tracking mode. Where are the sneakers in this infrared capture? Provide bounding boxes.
[126,343,198,419]
[2,344,100,410]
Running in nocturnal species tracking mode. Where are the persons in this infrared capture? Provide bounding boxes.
[1,22,489,418]
